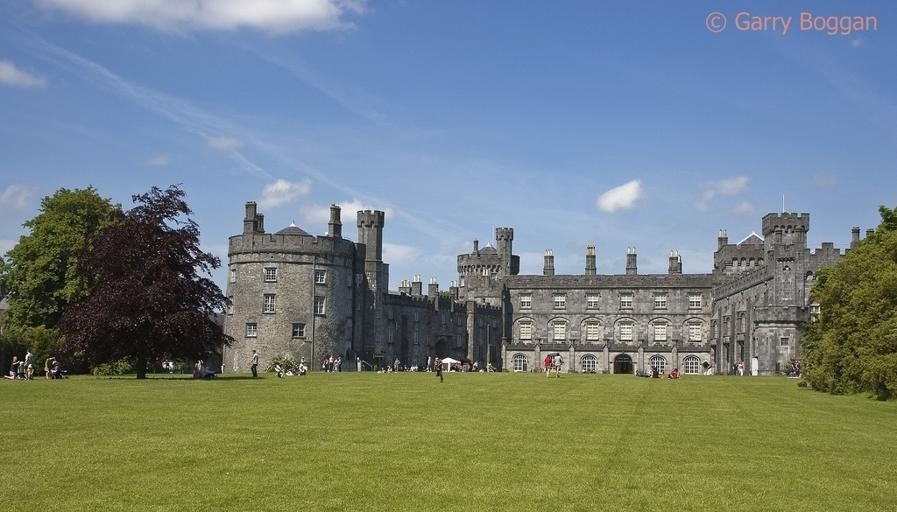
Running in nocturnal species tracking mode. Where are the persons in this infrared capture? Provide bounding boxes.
[277,369,285,378]
[667,368,677,379]
[737,362,745,376]
[297,363,305,376]
[732,362,739,376]
[322,354,342,373]
[377,352,492,382]
[786,358,799,377]
[543,355,552,377]
[674,368,680,378]
[298,357,305,369]
[192,366,201,378]
[4,348,64,380]
[553,353,563,377]
[285,369,294,376]
[200,365,215,380]
[250,350,258,377]
[650,368,659,378]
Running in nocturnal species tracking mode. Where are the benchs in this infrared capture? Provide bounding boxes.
[52,370,67,379]
[193,370,215,380]
